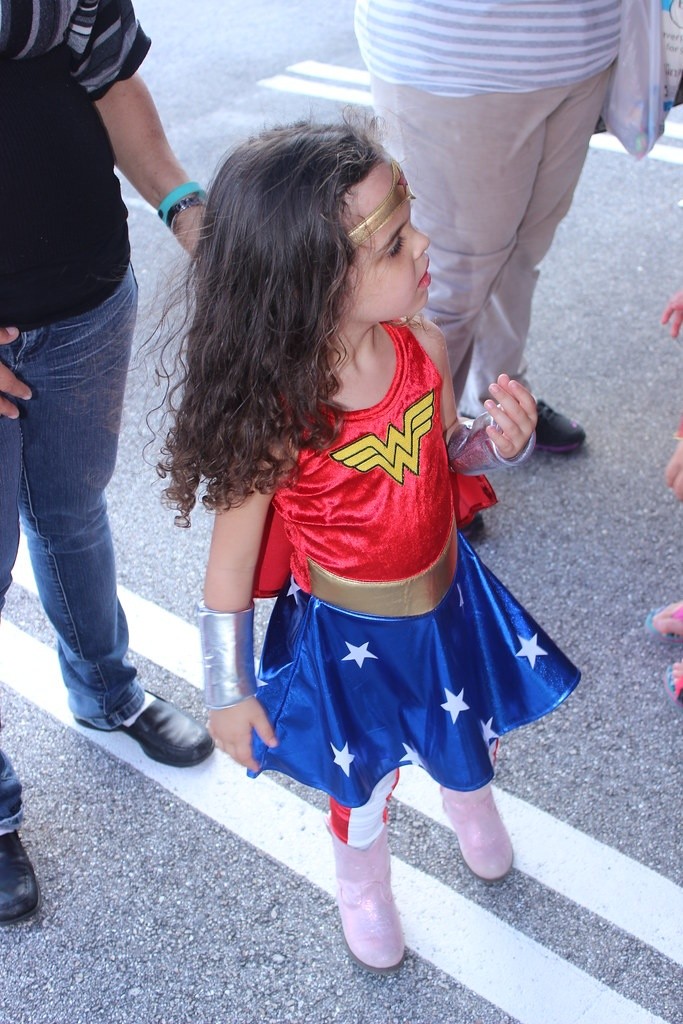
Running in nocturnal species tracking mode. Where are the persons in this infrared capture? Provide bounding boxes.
[645,289,682,704]
[140,106,582,973]
[354,0,621,542]
[1,0,215,923]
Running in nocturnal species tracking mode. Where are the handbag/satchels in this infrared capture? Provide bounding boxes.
[602,0,683,161]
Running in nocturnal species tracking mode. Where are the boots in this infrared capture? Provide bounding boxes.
[439,784,513,880]
[325,816,405,973]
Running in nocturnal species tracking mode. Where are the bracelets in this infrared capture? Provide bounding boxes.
[167,196,206,232]
[158,182,206,225]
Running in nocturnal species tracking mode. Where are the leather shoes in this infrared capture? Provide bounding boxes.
[0,829,43,927]
[73,685,215,768]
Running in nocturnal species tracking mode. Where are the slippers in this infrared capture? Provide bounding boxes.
[645,603,683,644]
[663,662,683,708]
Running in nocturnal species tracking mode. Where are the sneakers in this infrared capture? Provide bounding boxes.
[531,399,586,452]
